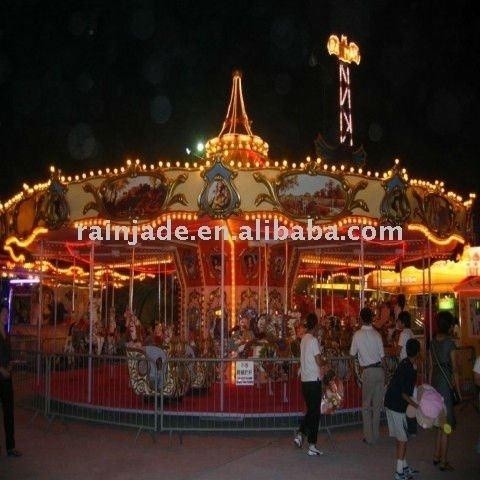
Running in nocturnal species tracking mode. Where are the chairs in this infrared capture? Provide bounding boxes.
[392,466,420,480]
[294,435,303,449]
[307,449,324,457]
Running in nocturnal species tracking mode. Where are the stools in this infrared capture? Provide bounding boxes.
[364,362,382,370]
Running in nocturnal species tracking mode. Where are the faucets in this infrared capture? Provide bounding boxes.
[433,454,457,471]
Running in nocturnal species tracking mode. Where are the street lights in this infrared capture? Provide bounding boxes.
[451,388,462,404]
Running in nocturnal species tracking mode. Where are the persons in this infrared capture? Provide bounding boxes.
[1,300,26,458]
[382,336,423,480]
[394,313,425,438]
[293,311,327,455]
[351,307,386,447]
[428,311,460,471]
